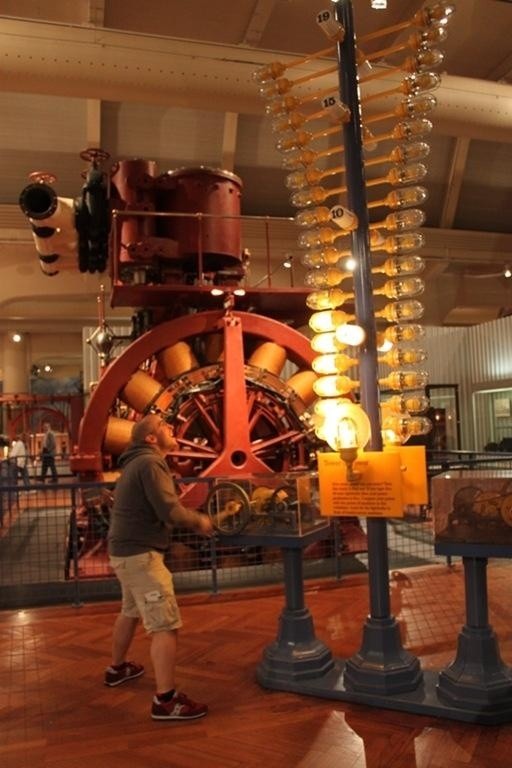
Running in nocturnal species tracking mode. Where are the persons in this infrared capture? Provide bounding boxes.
[2,432,37,495]
[37,423,58,483]
[103,413,216,720]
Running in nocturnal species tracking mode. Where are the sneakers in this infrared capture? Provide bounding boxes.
[103,661,145,687]
[13,478,58,496]
[150,689,209,720]
